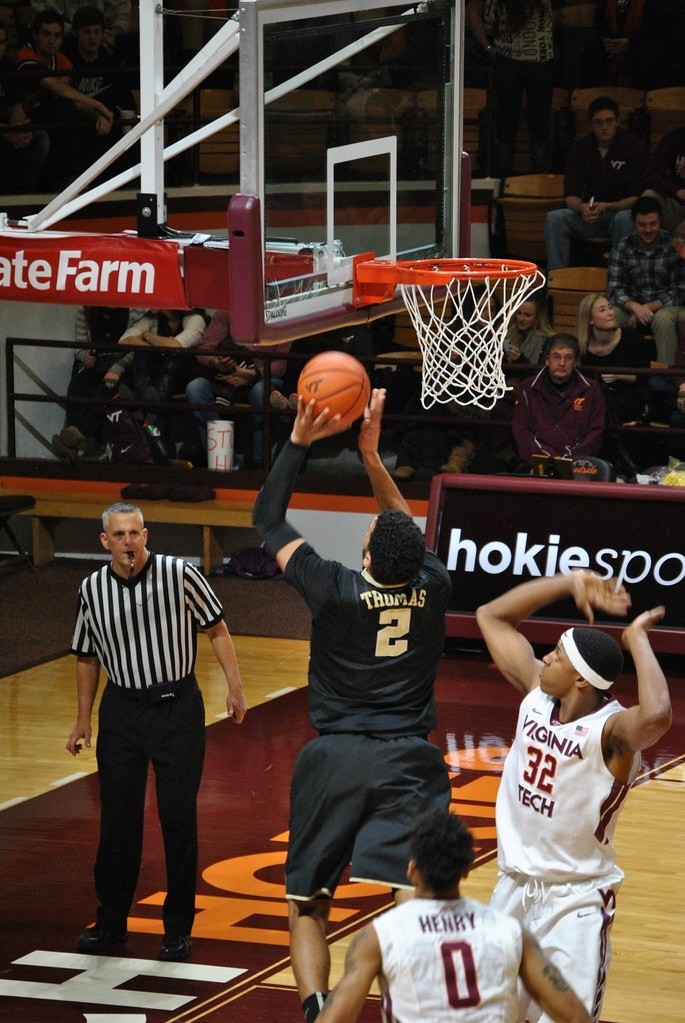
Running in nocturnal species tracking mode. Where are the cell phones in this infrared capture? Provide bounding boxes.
[589,197,595,211]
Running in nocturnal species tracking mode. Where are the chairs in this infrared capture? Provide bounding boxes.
[0,69,685,170]
[483,169,685,490]
[0,494,33,574]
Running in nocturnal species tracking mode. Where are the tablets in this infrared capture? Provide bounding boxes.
[532,454,574,481]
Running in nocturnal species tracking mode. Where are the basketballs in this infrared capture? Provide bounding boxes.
[296,349,372,423]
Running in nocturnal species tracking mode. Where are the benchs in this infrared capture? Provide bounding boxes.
[18,488,255,575]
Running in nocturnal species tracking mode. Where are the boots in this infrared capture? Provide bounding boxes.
[439,445,470,473]
[393,464,413,479]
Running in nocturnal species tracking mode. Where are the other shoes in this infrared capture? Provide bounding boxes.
[61,426,94,451]
[51,432,82,464]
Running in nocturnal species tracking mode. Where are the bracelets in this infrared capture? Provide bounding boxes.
[146,333,152,341]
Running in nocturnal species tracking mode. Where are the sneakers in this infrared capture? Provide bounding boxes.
[302,990,326,1023]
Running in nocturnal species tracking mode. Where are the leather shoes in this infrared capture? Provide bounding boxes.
[78,925,129,952]
[159,935,191,961]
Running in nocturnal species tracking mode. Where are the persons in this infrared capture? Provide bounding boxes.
[475,567,673,1023]
[312,810,594,1023]
[251,388,452,1023]
[0,0,685,476]
[64,503,247,961]
